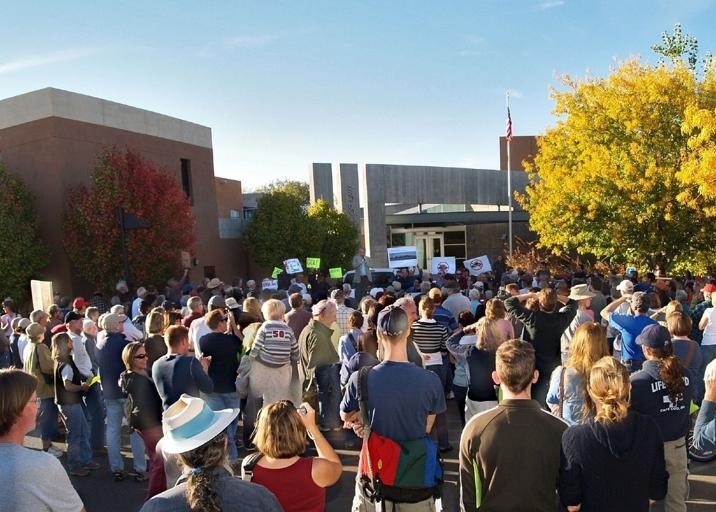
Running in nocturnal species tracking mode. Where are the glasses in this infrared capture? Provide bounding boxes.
[134,353,149,359]
[28,397,41,407]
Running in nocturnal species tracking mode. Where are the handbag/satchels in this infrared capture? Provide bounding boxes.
[35,343,55,384]
[358,364,445,503]
[559,367,566,417]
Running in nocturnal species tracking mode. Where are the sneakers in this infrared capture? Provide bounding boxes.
[43,448,63,457]
[113,470,129,481]
[134,471,149,480]
[81,462,101,469]
[50,443,63,452]
[69,468,89,476]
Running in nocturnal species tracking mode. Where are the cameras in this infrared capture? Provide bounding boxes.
[292,407,307,419]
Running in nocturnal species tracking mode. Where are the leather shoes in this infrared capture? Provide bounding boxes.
[439,445,454,452]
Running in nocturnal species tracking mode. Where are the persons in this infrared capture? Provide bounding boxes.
[156,290,196,327]
[644,269,716,307]
[441,280,471,324]
[132,294,157,333]
[330,289,356,336]
[142,313,169,377]
[8,318,23,368]
[59,297,72,314]
[338,311,365,361]
[339,306,447,511]
[394,298,424,368]
[667,311,703,472]
[258,283,311,311]
[600,291,660,373]
[690,285,716,346]
[200,310,243,465]
[17,319,30,368]
[243,297,264,322]
[85,307,100,321]
[187,295,230,359]
[23,323,62,458]
[610,279,634,359]
[469,289,480,314]
[352,247,373,299]
[30,311,52,346]
[452,311,477,427]
[390,267,423,288]
[341,284,357,309]
[357,303,385,366]
[477,298,514,341]
[152,324,213,490]
[118,342,164,502]
[649,301,683,329]
[626,324,693,512]
[559,284,594,366]
[241,400,343,512]
[236,322,301,450]
[562,356,670,512]
[65,311,107,455]
[291,273,306,294]
[245,279,258,298]
[1,299,21,338]
[165,270,212,306]
[410,297,454,453]
[284,293,313,336]
[546,321,610,424]
[507,284,518,294]
[493,255,505,283]
[504,288,578,412]
[52,333,101,475]
[208,278,223,296]
[0,368,86,512]
[298,300,340,447]
[49,306,63,326]
[504,266,644,290]
[0,331,8,369]
[379,295,394,305]
[73,297,88,313]
[224,297,241,324]
[113,305,143,342]
[147,284,158,296]
[600,297,619,356]
[231,277,243,301]
[430,269,498,299]
[80,319,96,335]
[132,287,147,317]
[139,393,284,512]
[372,278,432,302]
[116,281,133,319]
[692,358,716,450]
[529,288,564,311]
[699,292,716,364]
[446,317,507,425]
[95,314,148,481]
[183,296,204,328]
[459,339,578,512]
[308,268,331,300]
[249,298,300,368]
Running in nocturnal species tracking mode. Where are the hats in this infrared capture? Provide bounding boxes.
[104,314,128,323]
[429,288,442,299]
[65,311,83,323]
[157,393,241,454]
[26,323,46,336]
[700,284,716,292]
[635,323,672,348]
[18,318,32,329]
[567,283,596,300]
[616,280,634,291]
[73,298,90,308]
[208,278,224,289]
[379,305,409,336]
[225,297,242,309]
[472,281,484,289]
[331,289,346,299]
